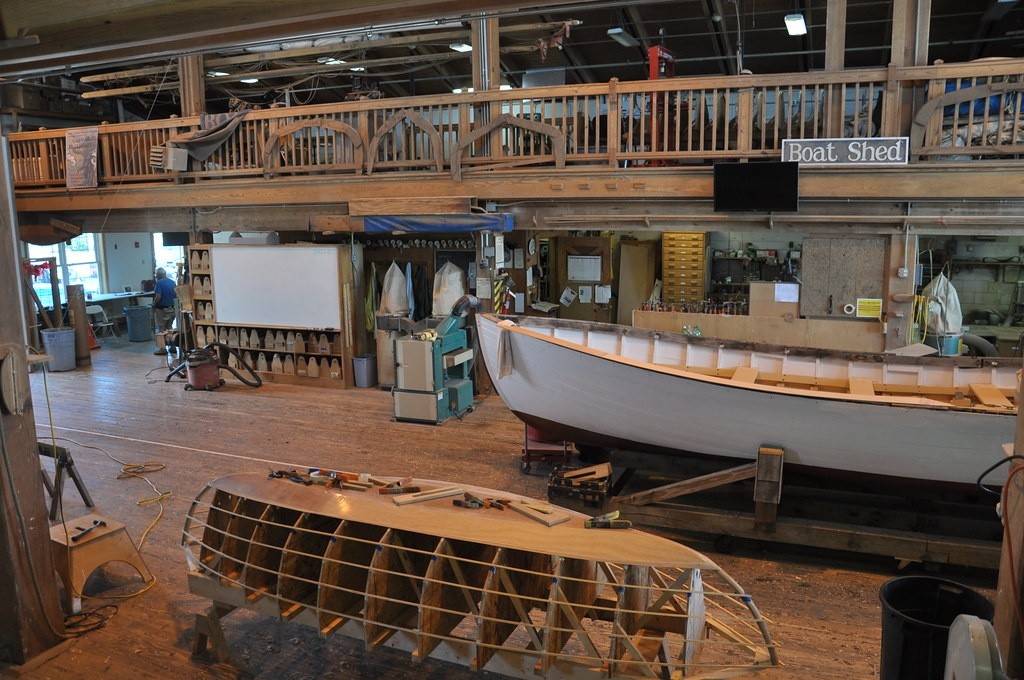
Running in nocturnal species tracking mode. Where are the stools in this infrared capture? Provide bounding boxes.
[50,514,153,614]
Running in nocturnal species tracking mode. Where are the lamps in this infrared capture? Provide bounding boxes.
[449,42,473,53]
[317,57,364,72]
[785,14,807,36]
[607,28,640,48]
[209,72,258,83]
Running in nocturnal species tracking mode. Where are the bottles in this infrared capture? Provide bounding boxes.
[641,299,748,316]
[680,324,702,336]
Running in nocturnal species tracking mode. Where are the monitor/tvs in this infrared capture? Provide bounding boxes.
[714,161,798,212]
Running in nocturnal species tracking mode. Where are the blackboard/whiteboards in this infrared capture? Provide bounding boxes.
[210,246,343,332]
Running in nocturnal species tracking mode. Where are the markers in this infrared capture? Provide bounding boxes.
[306,327,322,330]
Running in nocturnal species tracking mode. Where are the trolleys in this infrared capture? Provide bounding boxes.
[520,421,573,475]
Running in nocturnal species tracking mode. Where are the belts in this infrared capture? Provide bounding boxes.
[156,305,174,308]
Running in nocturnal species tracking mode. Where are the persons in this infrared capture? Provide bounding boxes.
[154,268,177,354]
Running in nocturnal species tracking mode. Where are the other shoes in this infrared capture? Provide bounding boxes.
[154,348,167,355]
[169,348,177,353]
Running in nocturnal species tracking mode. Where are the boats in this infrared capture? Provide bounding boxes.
[474,309,1023,488]
[171,468,784,677]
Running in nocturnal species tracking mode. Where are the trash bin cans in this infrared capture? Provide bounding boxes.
[124,305,153,341]
[39,327,77,373]
[877,575,995,680]
[353,353,377,388]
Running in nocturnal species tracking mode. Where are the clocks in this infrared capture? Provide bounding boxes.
[529,239,536,255]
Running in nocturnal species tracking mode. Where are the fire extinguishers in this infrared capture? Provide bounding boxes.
[502,286,512,315]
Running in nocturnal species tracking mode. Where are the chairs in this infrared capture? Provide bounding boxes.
[85,305,120,345]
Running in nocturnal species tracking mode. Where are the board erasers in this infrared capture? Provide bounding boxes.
[324,327,333,331]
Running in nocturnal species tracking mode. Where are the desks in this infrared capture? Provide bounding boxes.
[36,290,155,343]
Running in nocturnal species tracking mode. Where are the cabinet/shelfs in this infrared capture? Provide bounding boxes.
[661,231,789,309]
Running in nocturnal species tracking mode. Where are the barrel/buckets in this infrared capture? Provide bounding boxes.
[880,576,994,680]
